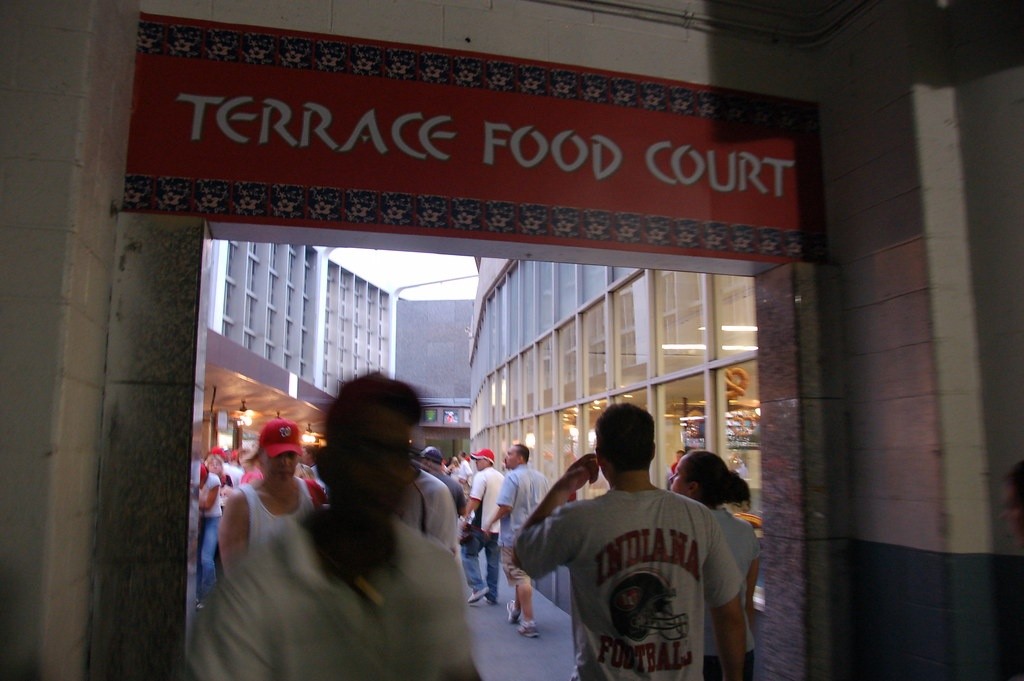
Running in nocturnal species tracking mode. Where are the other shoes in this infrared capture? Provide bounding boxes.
[482,596,497,604]
[197,602,205,608]
[468,587,489,603]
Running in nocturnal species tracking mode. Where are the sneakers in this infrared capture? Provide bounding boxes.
[506,600,521,624]
[517,621,539,638]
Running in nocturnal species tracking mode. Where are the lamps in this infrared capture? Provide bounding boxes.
[236,400,252,426]
[301,424,316,442]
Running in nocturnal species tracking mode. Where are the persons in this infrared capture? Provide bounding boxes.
[667,445,746,494]
[667,450,759,681]
[520,404,742,681]
[189,369,551,681]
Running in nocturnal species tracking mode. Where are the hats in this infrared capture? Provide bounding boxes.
[470,448,494,465]
[232,450,238,458]
[419,446,442,463]
[259,418,303,457]
[201,463,207,479]
[212,447,226,458]
